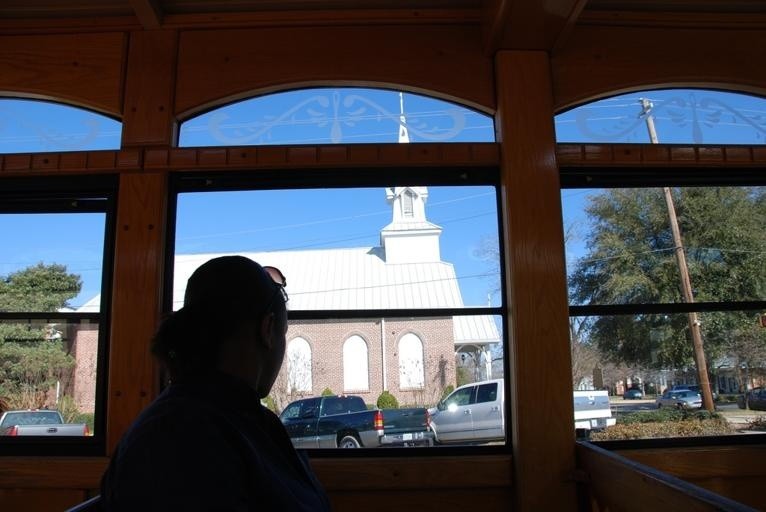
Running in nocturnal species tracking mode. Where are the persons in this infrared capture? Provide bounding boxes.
[98,257,330,512]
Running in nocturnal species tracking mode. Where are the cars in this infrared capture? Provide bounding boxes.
[736,387,766,412]
[656,389,702,411]
[623,390,642,399]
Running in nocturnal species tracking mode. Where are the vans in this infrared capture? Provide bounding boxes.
[664,385,718,402]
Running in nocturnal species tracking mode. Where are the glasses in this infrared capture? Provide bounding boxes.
[264,266,287,309]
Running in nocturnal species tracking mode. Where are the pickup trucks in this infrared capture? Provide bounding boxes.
[279,396,429,448]
[0,410,90,436]
[428,379,616,445]
